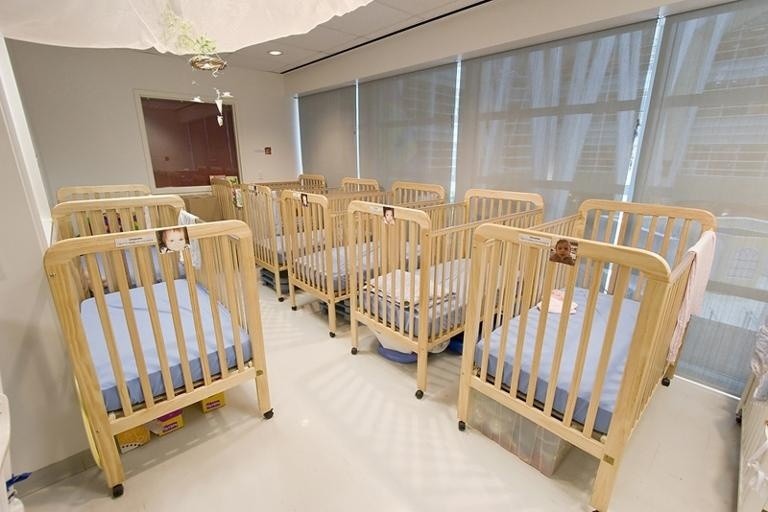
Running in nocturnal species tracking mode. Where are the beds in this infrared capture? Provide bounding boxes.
[46,185,274,498]
[347,188,545,399]
[212,175,327,222]
[280,183,446,338]
[243,177,382,303]
[458,200,718,512]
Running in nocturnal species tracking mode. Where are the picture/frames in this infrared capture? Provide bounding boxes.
[133,89,244,195]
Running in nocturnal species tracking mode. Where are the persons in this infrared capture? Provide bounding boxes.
[300,194,308,207]
[549,237,579,265]
[382,206,395,224]
[155,227,190,256]
